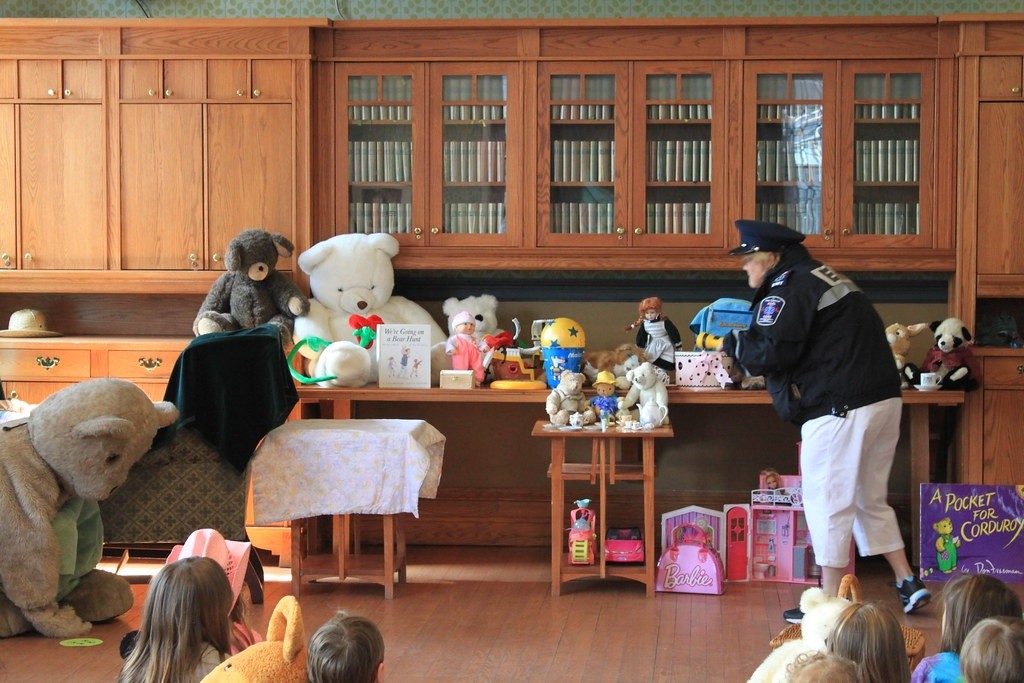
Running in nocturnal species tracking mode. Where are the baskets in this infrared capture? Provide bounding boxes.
[770,574,926,674]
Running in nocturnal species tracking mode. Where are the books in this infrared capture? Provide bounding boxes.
[349,76,505,234]
[552,74,711,233]
[757,77,919,234]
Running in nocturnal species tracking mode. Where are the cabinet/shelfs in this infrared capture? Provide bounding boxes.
[0,13,1024,574]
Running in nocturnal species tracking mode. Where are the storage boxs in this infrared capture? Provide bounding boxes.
[675,352,733,387]
[440,370,475,389]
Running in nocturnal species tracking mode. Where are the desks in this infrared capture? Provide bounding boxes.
[296,385,965,568]
[533,421,673,598]
[252,419,446,599]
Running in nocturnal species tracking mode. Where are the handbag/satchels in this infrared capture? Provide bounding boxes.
[689,297,753,352]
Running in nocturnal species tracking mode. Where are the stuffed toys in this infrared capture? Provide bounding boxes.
[0,378,180,638]
[623,362,669,426]
[924,318,980,391]
[192,229,311,346]
[885,322,927,370]
[293,232,448,388]
[591,370,619,419]
[201,596,309,683]
[545,370,596,425]
[746,587,852,683]
[430,294,505,384]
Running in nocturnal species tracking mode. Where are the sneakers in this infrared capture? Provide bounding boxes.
[783,608,805,624]
[896,575,932,613]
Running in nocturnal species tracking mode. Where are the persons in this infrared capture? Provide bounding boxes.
[717,220,930,624]
[166,529,262,655]
[445,312,485,387]
[117,556,234,683]
[787,574,1024,683]
[306,612,383,683]
[625,298,683,370]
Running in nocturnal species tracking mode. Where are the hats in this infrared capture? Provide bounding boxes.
[0,309,64,338]
[728,220,806,256]
[166,529,251,616]
